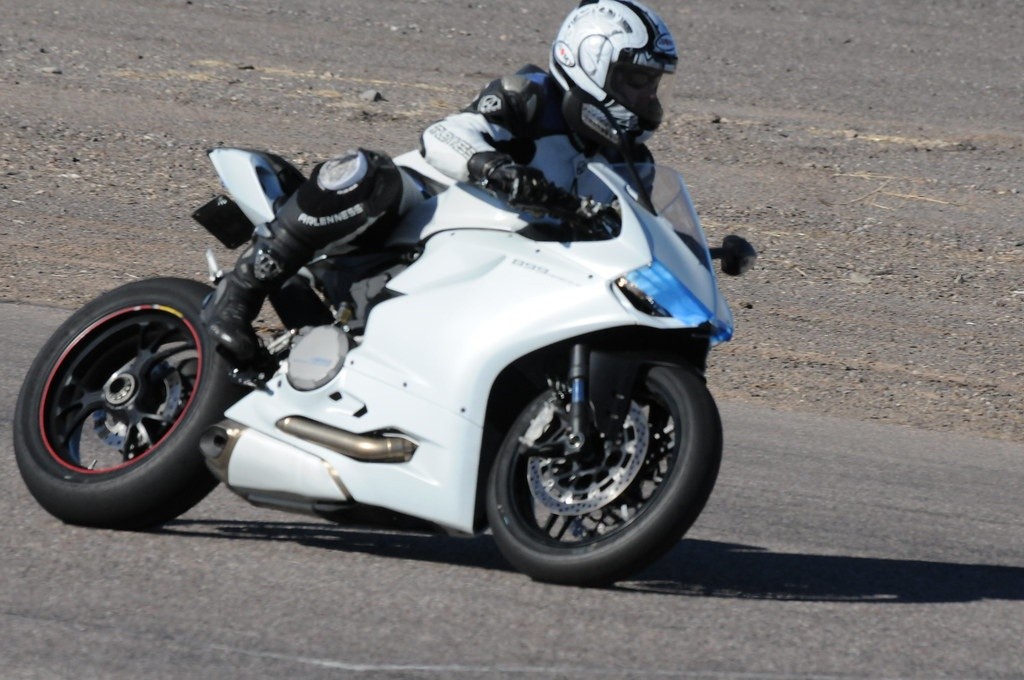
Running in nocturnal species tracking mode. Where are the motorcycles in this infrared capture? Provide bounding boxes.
[12,89,760,590]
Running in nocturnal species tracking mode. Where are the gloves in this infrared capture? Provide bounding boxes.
[467,151,548,206]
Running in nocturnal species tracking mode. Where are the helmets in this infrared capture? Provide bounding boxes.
[553,0,677,144]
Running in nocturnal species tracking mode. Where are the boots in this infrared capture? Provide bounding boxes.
[199,190,328,353]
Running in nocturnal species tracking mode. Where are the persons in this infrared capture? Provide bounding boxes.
[195,0,679,362]
